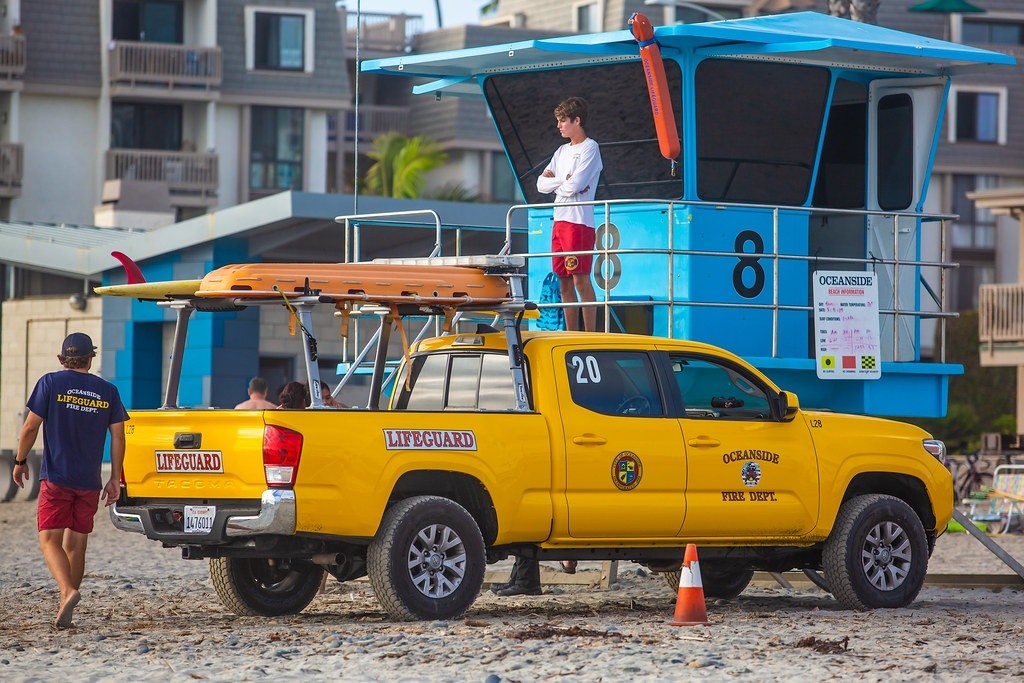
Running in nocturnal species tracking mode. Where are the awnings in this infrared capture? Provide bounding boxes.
[359,39,684,100]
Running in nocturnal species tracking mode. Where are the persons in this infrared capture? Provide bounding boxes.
[277,378,351,409]
[535,96,603,332]
[233,377,279,410]
[12,333,126,630]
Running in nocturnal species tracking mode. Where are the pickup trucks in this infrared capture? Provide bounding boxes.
[104,299,961,603]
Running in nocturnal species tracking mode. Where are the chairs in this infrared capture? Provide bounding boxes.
[944,432,1024,538]
[566,364,662,415]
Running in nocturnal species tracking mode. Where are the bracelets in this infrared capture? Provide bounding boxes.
[14,455,27,466]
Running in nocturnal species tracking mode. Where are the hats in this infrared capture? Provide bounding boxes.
[62,332,97,356]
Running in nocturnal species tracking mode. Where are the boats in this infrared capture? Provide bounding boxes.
[192,260,514,307]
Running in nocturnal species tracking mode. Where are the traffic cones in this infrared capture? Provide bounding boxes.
[667,544,721,629]
